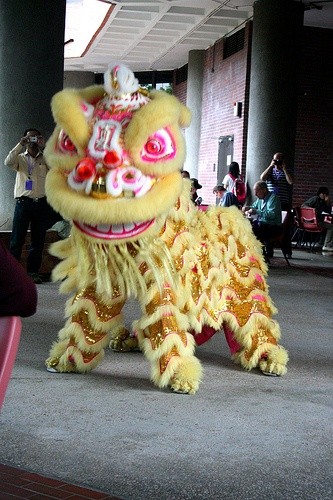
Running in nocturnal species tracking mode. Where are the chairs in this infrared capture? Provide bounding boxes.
[290,207,323,250]
[257,211,292,268]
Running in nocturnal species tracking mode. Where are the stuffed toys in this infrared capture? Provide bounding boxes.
[43,65,290,395]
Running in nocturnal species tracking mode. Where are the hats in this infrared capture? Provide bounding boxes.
[190,178,202,189]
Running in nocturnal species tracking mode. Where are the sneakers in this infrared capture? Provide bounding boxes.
[322,245,333,250]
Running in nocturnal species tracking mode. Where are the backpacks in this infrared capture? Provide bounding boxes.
[228,172,247,204]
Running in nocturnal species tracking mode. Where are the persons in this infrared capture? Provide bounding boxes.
[301,186,333,252]
[180,170,203,206]
[0,239,39,318]
[246,183,283,250]
[212,184,240,207]
[261,152,293,259]
[4,128,46,273]
[221,161,246,207]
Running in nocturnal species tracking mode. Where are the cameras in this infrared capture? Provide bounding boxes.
[274,160,283,164]
[27,136,38,143]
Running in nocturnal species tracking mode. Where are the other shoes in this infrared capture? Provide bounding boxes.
[28,273,42,284]
[286,253,291,259]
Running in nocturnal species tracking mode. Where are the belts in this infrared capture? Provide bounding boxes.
[18,198,44,201]
